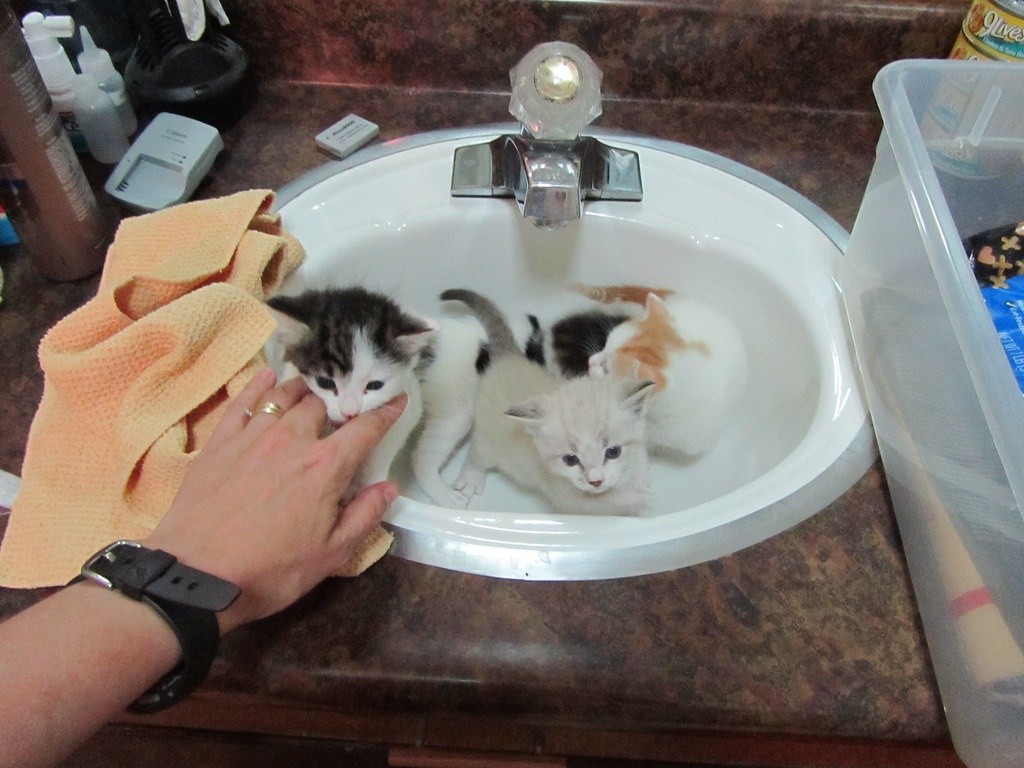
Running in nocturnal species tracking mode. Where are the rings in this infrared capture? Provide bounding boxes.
[250,402,285,418]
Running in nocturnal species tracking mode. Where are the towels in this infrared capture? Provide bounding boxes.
[0,186,397,592]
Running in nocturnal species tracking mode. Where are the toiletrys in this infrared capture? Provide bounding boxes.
[1,10,139,282]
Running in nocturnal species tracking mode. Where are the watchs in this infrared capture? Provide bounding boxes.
[63,539,241,717]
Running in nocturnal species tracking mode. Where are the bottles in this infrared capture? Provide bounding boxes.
[77,23,137,141]
[919,2,1024,182]
[70,74,128,165]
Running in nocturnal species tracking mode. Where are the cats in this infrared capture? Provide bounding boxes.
[261,287,744,518]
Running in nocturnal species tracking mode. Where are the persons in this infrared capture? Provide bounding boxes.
[1,366,407,768]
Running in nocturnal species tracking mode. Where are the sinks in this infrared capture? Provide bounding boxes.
[271,207,879,583]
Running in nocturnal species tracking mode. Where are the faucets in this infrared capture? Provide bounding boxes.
[449,40,643,231]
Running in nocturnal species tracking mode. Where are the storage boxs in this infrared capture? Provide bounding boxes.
[841,57,1022,767]
